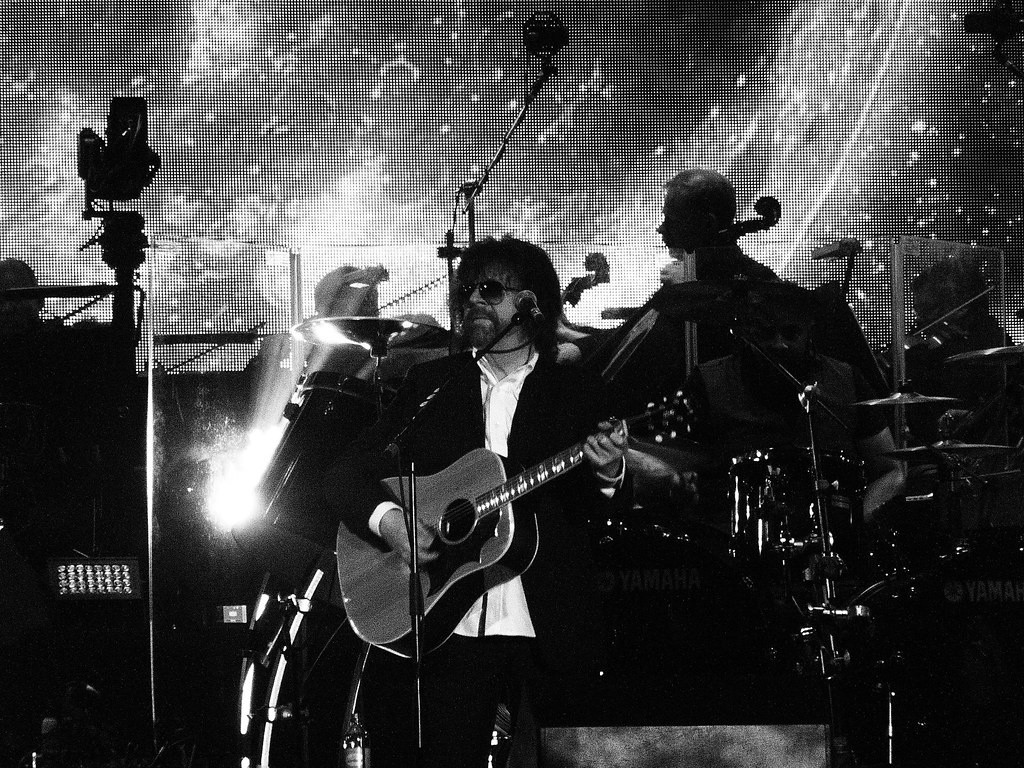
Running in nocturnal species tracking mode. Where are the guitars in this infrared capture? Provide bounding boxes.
[334,385,701,664]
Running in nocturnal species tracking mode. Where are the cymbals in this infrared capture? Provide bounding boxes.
[943,343,1024,369]
[311,311,457,362]
[845,390,963,411]
[882,438,1018,463]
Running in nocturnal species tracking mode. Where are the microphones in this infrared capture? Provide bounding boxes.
[514,290,545,325]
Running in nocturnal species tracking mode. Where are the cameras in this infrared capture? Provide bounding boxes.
[522,9,570,55]
[77,96,163,202]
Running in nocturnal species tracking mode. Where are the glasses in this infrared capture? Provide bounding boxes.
[456,277,523,306]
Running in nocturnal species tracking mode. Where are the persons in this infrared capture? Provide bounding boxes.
[1,256,75,327]
[869,258,1017,431]
[317,233,637,767]
[654,167,785,290]
